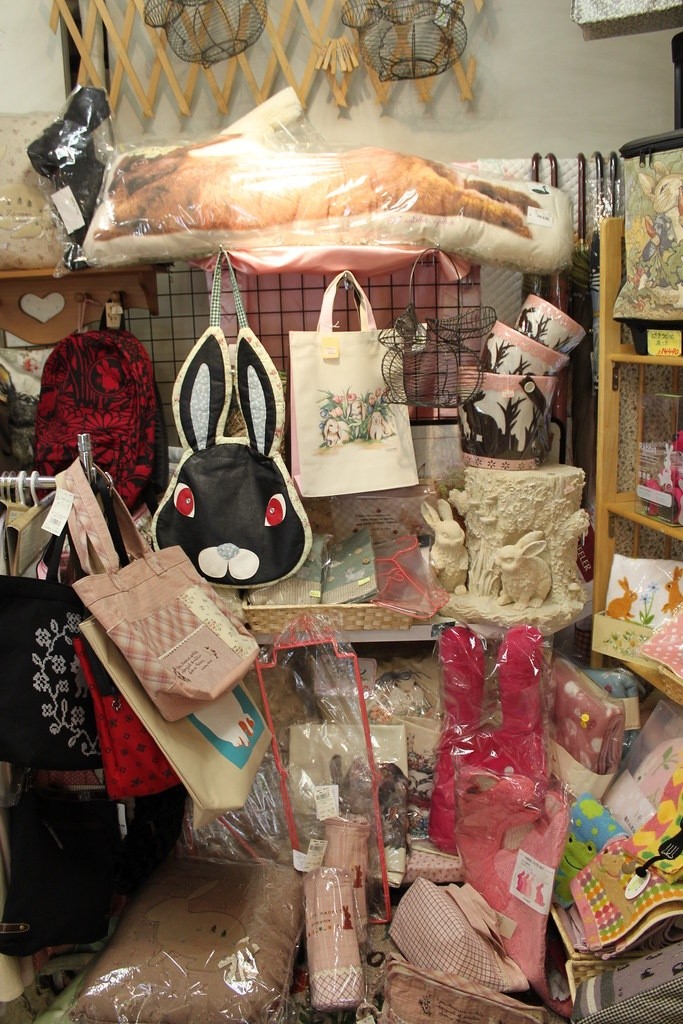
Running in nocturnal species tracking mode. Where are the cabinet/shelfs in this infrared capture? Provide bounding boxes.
[589,220,683,703]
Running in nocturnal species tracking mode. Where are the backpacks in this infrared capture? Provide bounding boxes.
[33,294,164,511]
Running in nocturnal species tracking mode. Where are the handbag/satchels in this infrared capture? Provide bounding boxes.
[390,878,530,992]
[356,951,548,1023]
[148,244,314,588]
[290,268,421,499]
[0,478,275,827]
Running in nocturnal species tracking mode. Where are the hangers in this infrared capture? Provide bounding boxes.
[0,470,49,506]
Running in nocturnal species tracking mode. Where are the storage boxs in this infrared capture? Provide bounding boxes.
[593,609,661,670]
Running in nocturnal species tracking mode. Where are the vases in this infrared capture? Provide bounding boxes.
[457,294,583,474]
